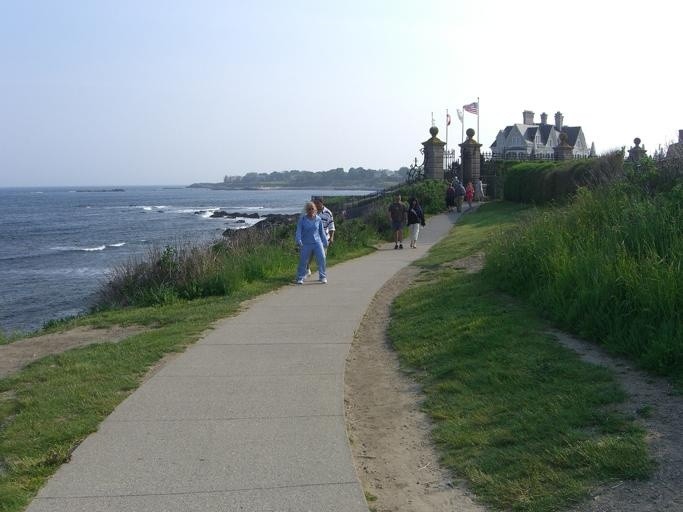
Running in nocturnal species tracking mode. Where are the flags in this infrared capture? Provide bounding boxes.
[455,106,464,123]
[445,113,451,127]
[463,102,479,115]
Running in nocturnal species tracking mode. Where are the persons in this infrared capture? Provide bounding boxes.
[407,196,426,249]
[446,183,455,213]
[386,192,406,250]
[293,199,329,285]
[456,181,466,213]
[303,195,336,279]
[474,175,484,205]
[463,182,475,209]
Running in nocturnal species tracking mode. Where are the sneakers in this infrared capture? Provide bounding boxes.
[297,271,311,284]
[322,278,327,283]
